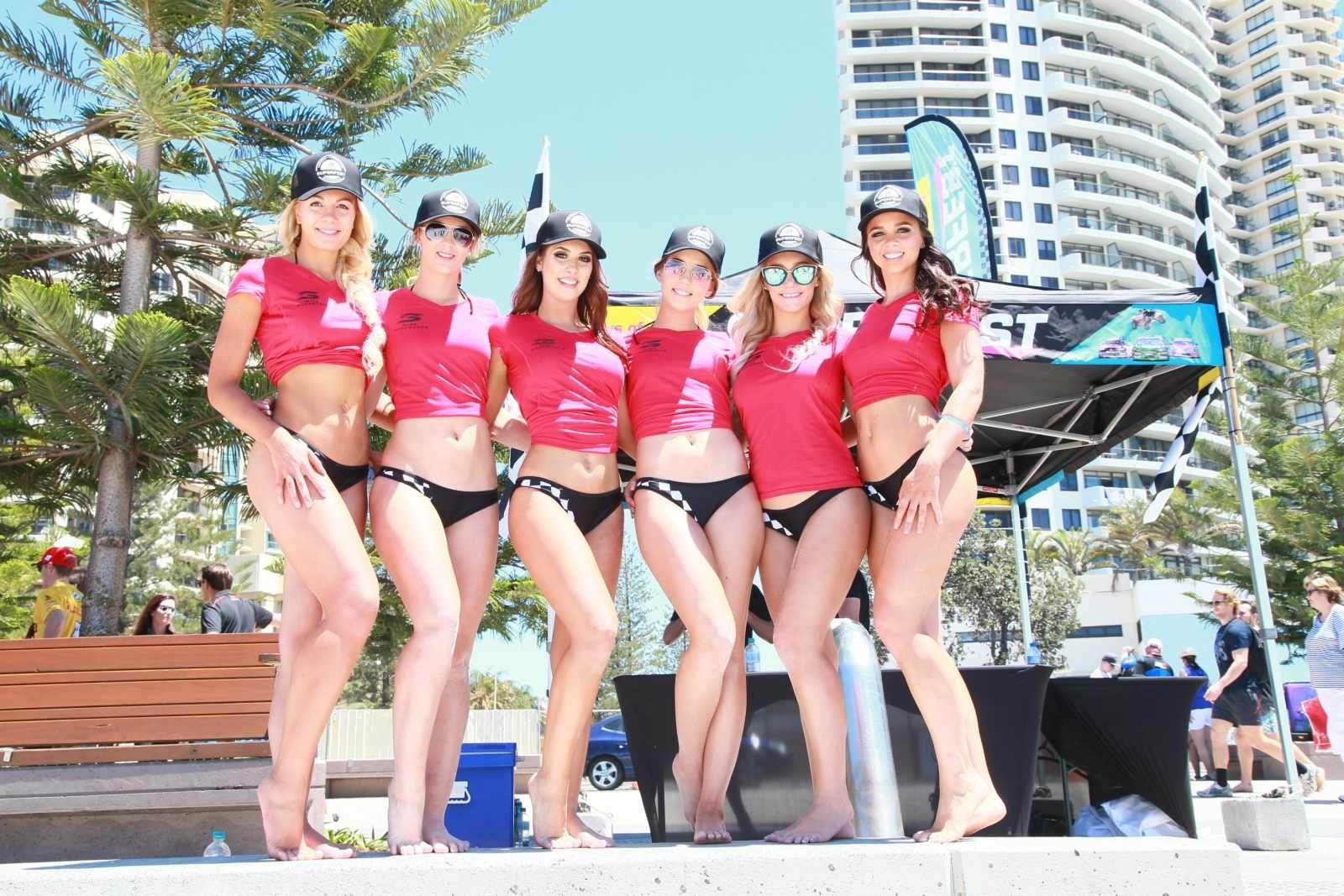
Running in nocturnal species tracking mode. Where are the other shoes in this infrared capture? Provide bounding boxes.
[1298,763,1318,797]
[1197,782,1233,798]
[1195,775,1215,780]
[1232,781,1253,792]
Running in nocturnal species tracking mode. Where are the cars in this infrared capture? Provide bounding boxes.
[583,712,788,791]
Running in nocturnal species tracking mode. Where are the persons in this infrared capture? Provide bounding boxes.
[1091,570,1344,803]
[201,562,274,634]
[30,546,84,638]
[132,593,177,634]
[207,151,1008,861]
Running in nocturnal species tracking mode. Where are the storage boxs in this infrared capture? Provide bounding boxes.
[445,742,517,846]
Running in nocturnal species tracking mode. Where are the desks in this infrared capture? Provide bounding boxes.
[1038,676,1209,838]
[612,666,1056,843]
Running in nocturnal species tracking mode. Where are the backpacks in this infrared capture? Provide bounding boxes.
[1222,618,1269,683]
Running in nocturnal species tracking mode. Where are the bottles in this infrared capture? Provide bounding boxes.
[1026,642,1041,665]
[202,830,231,857]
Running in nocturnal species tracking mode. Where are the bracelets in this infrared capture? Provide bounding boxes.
[940,414,968,432]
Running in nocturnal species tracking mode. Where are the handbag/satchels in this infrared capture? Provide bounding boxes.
[1072,792,1189,837]
[1301,697,1332,754]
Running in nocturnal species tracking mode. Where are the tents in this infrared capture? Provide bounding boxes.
[604,225,1302,800]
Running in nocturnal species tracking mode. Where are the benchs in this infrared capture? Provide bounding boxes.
[325,708,543,798]
[0,633,280,766]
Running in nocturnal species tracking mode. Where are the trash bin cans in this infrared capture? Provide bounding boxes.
[444,743,515,849]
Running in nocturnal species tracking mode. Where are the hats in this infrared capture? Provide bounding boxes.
[1180,648,1197,658]
[412,187,482,242]
[856,184,928,231]
[30,547,76,568]
[536,209,607,260]
[1102,654,1120,665]
[757,222,824,269]
[661,223,726,277]
[291,151,365,202]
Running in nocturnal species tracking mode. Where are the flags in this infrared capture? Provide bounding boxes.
[905,114,999,283]
[521,138,549,260]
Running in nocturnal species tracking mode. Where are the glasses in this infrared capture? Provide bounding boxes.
[662,258,713,286]
[1307,589,1318,596]
[1208,601,1228,606]
[760,264,823,288]
[421,222,475,249]
[157,605,176,612]
